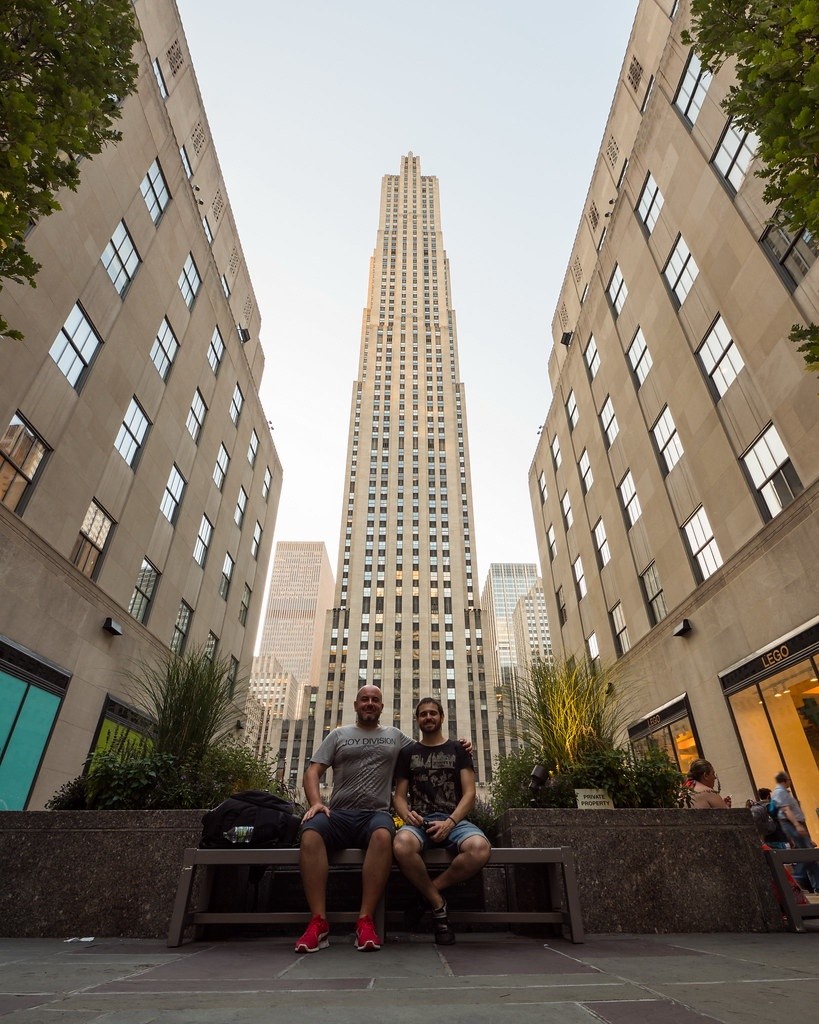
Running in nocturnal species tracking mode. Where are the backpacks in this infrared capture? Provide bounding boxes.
[750,802,777,832]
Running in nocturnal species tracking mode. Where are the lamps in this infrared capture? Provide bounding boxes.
[192,184,200,191]
[609,198,616,204]
[239,329,251,344]
[560,331,574,347]
[103,617,123,635]
[672,619,692,636]
[528,764,549,792]
[605,212,611,217]
[197,198,204,205]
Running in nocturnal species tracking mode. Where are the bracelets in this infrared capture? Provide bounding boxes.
[444,817,457,826]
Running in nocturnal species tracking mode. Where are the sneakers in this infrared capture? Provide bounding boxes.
[294,914,330,952]
[353,913,381,951]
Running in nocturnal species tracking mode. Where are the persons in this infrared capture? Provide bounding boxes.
[293,684,474,953]
[675,758,819,919]
[391,698,491,933]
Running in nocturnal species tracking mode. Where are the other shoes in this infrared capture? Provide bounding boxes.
[429,893,456,945]
[404,896,429,928]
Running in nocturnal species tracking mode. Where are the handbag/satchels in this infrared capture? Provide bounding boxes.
[199,797,303,848]
[769,799,776,812]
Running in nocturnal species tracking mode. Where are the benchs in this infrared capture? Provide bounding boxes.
[763,848,819,934]
[166,848,585,948]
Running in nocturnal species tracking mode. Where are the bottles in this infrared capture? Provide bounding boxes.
[223,826,254,843]
